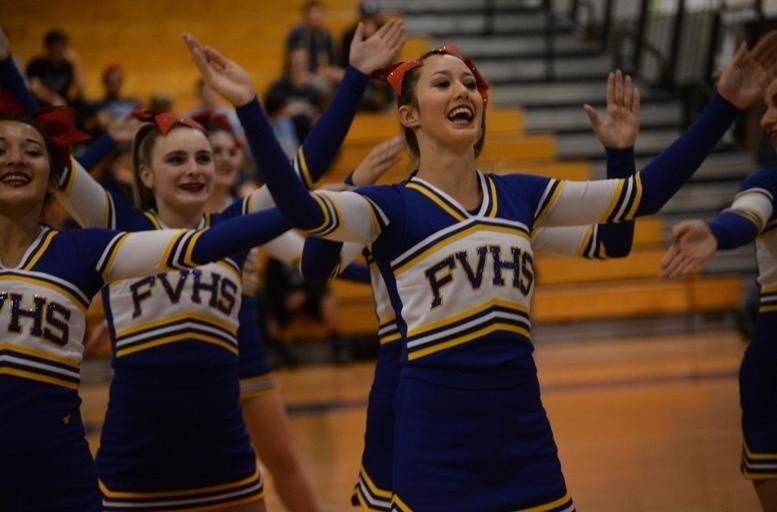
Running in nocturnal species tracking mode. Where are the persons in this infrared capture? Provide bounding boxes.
[660,75,777,512]
[180,30,777,512]
[760,77,777,145]
[192,111,374,512]
[0,107,404,512]
[297,70,640,512]
[0,16,407,512]
[22,0,396,336]
